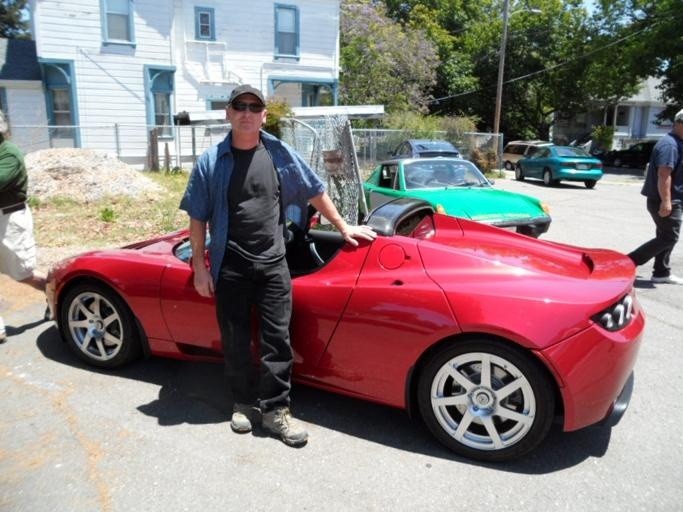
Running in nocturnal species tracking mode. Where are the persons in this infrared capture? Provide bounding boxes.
[628,107,682,285]
[177,83,377,447]
[0,110,52,346]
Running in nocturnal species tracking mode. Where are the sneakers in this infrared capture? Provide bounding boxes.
[650,273,683,287]
[231,402,255,433]
[261,408,309,447]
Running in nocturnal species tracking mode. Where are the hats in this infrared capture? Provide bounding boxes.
[227,84,265,105]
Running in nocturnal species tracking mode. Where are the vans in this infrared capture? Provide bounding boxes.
[503,139,553,170]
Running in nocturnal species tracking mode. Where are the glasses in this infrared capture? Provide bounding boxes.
[231,101,265,114]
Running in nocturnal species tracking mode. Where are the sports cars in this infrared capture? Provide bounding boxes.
[361,156,549,241]
[42,211,645,463]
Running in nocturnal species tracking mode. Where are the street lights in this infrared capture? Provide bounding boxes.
[492,9,542,152]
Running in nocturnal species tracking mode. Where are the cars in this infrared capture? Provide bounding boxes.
[515,145,603,190]
[388,138,466,184]
[610,140,657,169]
